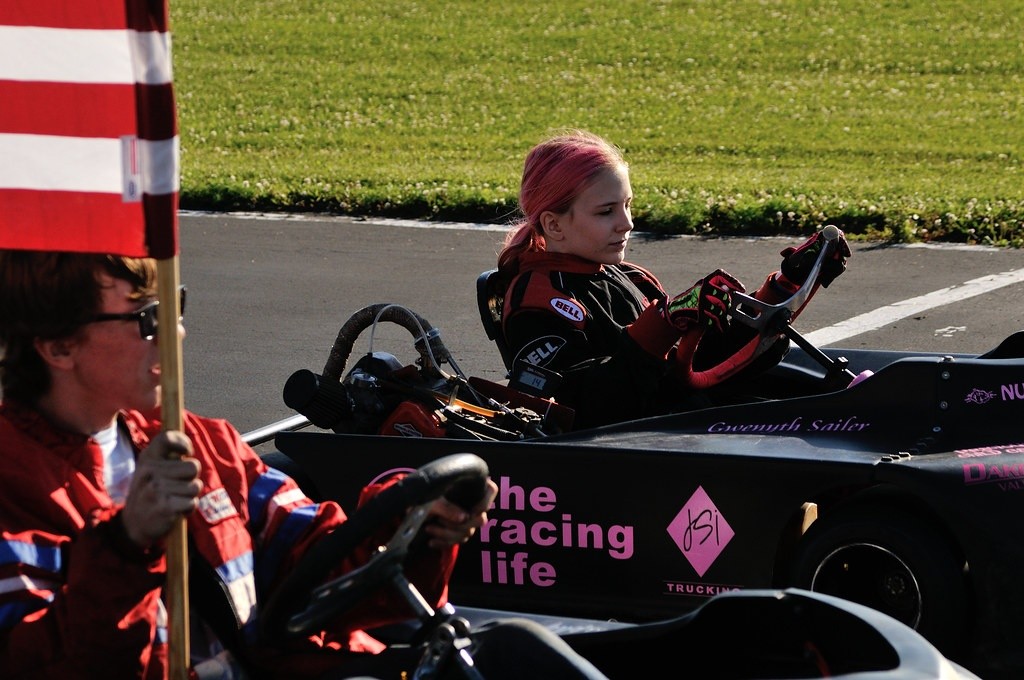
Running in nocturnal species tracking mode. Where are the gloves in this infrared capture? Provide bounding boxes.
[780,225,853,288]
[664,267,746,334]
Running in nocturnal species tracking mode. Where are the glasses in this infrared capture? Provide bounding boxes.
[76,284,188,340]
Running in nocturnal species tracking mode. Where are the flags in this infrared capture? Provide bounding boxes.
[0,0,178,256]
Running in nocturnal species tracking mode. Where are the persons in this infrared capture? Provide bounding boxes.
[498,136,851,431]
[0,249,498,680]
